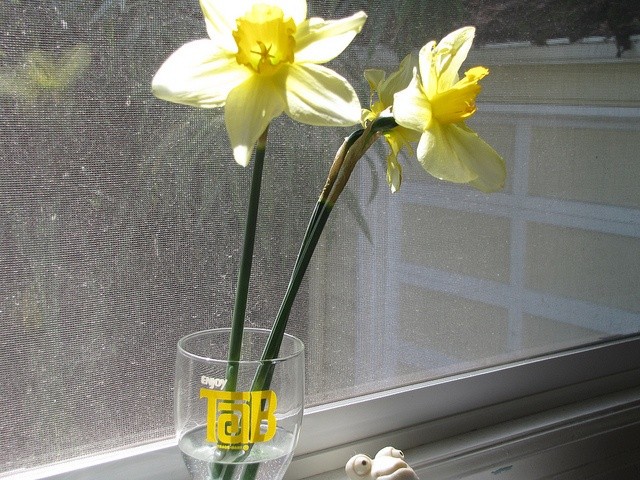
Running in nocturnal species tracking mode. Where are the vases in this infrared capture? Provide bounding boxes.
[174,325,306,480]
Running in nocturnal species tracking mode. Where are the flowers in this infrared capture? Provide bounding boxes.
[150,0,507,480]
